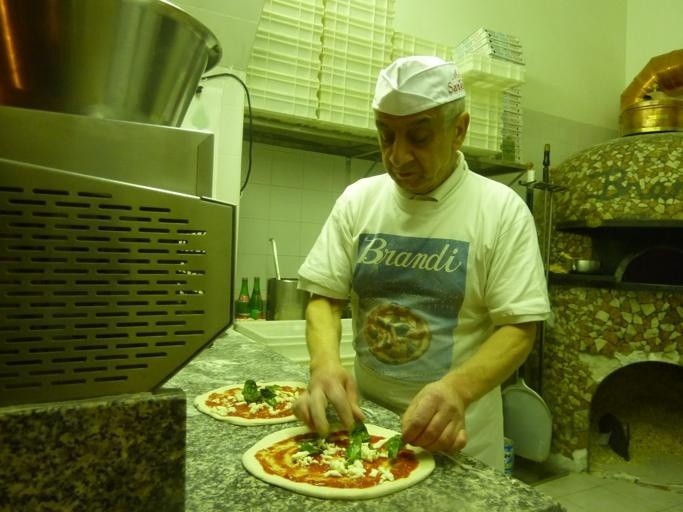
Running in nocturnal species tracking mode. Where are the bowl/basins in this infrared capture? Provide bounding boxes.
[0,0,223,127]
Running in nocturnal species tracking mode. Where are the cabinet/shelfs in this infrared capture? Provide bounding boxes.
[243,114,382,156]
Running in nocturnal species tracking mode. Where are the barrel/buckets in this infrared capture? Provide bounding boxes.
[265,276,311,321]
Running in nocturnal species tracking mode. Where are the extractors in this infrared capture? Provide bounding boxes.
[550,131,682,229]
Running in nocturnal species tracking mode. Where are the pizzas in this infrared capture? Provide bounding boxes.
[241,423,436,501]
[193,378,307,428]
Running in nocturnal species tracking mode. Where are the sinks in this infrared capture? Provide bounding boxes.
[233,318,354,341]
[267,342,356,366]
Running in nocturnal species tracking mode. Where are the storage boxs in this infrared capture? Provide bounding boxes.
[391,33,454,67]
[457,31,523,159]
[244,0,326,118]
[320,1,398,123]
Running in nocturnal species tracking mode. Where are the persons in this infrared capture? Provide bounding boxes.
[291,55,553,473]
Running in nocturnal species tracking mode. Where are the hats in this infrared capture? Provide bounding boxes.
[372,55,466,117]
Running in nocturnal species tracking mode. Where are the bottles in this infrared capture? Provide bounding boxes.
[235,276,264,320]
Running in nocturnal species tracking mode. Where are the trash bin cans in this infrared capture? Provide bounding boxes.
[503,436,515,475]
